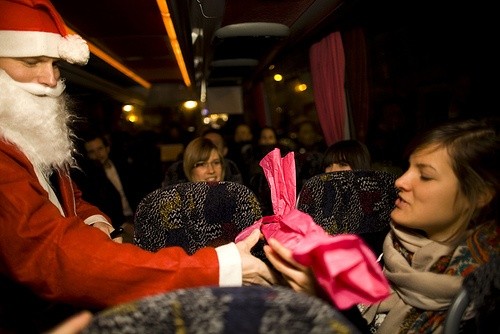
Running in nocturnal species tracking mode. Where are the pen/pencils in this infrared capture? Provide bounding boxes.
[110,228,124,239]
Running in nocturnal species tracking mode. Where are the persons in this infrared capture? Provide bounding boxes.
[182,136,227,184]
[83,134,144,238]
[1,0,280,334]
[161,128,243,184]
[321,139,371,175]
[263,117,500,334]
[76,101,326,166]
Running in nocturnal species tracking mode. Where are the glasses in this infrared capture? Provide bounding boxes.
[193,160,221,170]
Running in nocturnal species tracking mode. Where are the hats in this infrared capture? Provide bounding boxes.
[0,0,91,65]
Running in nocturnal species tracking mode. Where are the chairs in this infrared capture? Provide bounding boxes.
[293,169,403,244]
[133,181,261,256]
